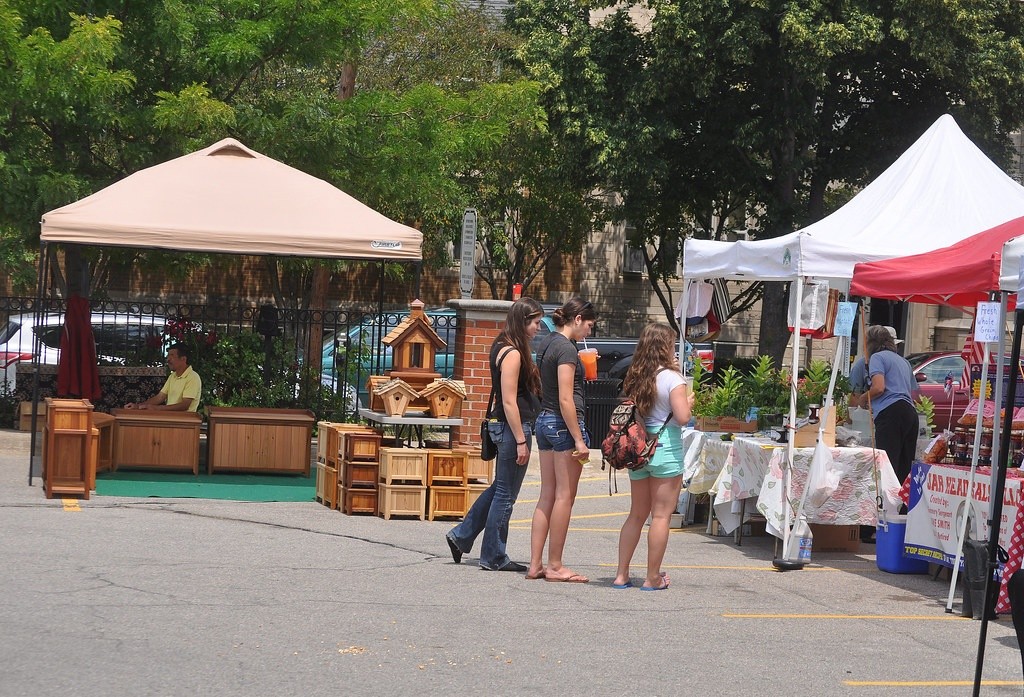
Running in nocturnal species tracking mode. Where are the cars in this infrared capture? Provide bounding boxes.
[898,351,1024,440]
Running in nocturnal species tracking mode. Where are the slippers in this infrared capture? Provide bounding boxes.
[524,568,547,579]
[546,569,589,584]
[640,575,669,591]
[612,577,633,590]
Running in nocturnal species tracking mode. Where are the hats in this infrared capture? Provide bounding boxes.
[882,326,906,345]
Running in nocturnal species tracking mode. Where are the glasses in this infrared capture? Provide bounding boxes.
[574,301,594,320]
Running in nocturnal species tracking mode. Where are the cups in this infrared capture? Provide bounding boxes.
[577,348,598,382]
[683,376,694,397]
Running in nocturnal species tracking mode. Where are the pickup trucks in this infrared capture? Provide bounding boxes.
[533,302,715,450]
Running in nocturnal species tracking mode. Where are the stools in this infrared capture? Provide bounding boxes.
[315,421,375,509]
[346,433,382,462]
[342,486,377,516]
[378,447,428,486]
[379,482,427,520]
[467,450,492,485]
[344,461,378,490]
[422,449,468,488]
[426,485,471,522]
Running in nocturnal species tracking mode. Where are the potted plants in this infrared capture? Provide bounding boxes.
[787,359,853,426]
[731,353,792,430]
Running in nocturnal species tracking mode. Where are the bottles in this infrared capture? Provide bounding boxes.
[786,515,814,564]
[770,413,790,443]
[948,426,1024,470]
[809,403,821,424]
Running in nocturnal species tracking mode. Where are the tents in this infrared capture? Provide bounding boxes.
[849,216,1024,610]
[28,137,423,486]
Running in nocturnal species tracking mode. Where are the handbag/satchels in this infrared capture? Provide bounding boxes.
[674,276,733,343]
[481,420,497,461]
[789,278,858,339]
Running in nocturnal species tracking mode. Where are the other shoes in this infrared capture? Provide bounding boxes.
[482,560,526,572]
[445,533,464,564]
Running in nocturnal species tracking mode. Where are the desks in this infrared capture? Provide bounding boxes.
[709,436,902,558]
[898,459,1024,615]
[357,407,464,448]
[680,426,787,545]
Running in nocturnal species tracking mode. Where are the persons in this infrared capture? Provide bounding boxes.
[860,325,920,544]
[680,114,1024,558]
[848,326,905,410]
[446,297,542,571]
[526,298,601,582]
[124,344,202,412]
[613,323,695,591]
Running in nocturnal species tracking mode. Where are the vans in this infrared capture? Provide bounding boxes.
[287,309,576,408]
[0,311,363,421]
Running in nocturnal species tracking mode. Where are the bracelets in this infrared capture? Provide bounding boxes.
[517,441,526,445]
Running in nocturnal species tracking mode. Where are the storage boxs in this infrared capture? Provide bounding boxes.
[648,510,684,529]
[794,405,837,447]
[694,415,757,432]
[810,521,860,552]
[874,509,929,574]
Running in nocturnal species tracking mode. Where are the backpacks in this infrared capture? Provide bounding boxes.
[601,365,674,471]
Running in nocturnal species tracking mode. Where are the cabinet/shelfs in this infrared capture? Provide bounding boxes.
[110,408,203,475]
[42,397,95,499]
[206,405,316,477]
